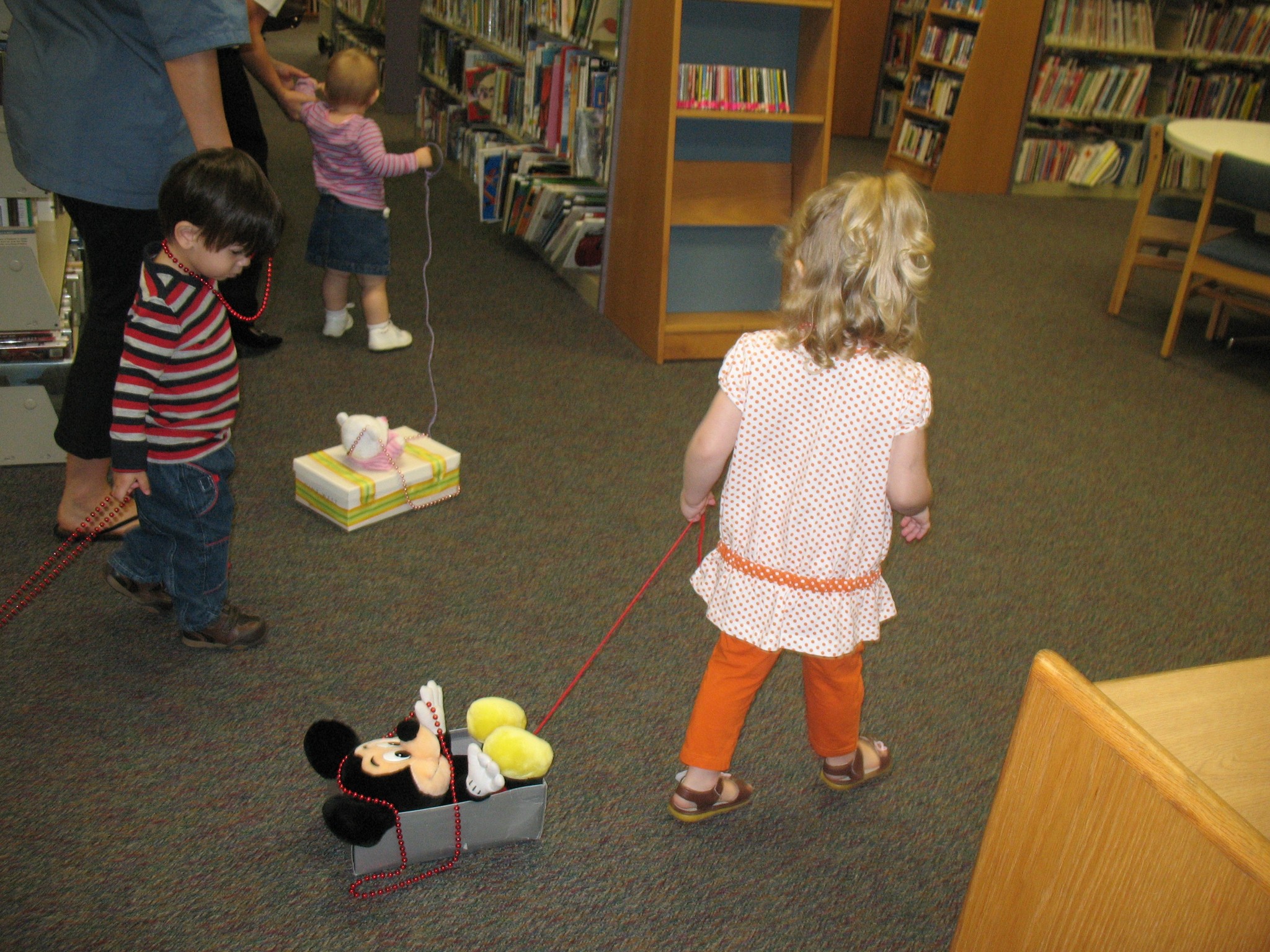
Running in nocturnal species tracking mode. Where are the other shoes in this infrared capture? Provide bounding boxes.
[231,326,283,358]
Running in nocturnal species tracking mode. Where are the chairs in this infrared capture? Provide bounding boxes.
[1158,151,1270,363]
[1107,112,1255,340]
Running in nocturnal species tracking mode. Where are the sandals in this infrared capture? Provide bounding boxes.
[666,770,749,822]
[819,735,891,790]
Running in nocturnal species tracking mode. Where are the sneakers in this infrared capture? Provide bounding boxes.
[103,562,174,605]
[368,320,413,351]
[321,313,356,337]
[180,601,266,650]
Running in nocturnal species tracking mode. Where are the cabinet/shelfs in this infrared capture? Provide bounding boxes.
[869,0,930,144]
[883,0,1045,199]
[325,0,423,114]
[410,0,623,312]
[0,1,84,467]
[1007,0,1270,201]
[606,0,841,365]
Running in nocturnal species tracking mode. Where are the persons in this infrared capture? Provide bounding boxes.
[667,172,944,824]
[0,0,433,650]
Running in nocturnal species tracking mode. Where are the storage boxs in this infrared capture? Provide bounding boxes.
[291,424,464,533]
[347,728,550,880]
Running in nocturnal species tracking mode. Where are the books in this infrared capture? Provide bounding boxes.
[869,1,1270,192]
[293,1,791,280]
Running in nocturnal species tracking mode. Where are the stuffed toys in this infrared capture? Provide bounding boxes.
[305,680,554,847]
[336,411,401,472]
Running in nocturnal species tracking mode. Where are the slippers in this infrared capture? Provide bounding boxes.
[54,493,141,541]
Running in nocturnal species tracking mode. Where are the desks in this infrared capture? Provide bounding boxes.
[1165,117,1270,353]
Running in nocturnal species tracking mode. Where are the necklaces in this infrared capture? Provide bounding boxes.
[162,236,273,320]
[336,700,462,899]
[346,424,461,509]
